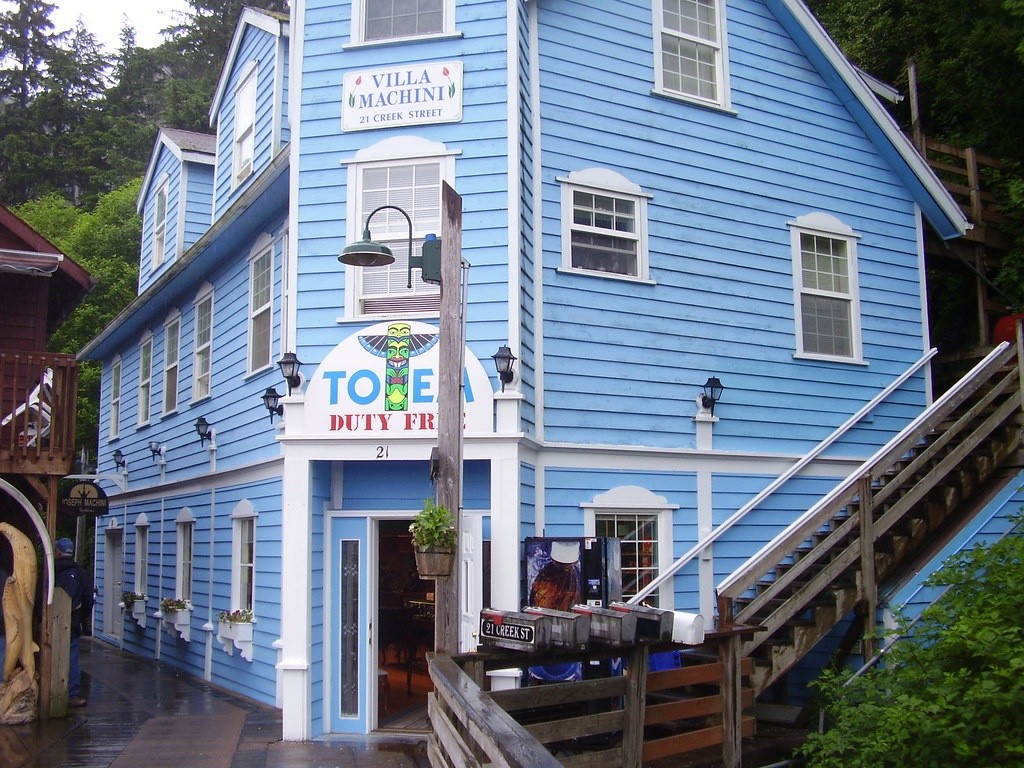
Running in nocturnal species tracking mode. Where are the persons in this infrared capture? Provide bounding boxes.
[54,538,92,707]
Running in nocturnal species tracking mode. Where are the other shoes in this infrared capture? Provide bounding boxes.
[69,696,87,707]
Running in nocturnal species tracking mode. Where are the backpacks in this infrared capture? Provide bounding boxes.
[58,565,84,611]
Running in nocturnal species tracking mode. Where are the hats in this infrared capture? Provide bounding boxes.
[55,538,73,554]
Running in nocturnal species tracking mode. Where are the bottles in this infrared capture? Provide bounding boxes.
[527,541,589,685]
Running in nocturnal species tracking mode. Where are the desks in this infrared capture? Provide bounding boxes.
[407,615,435,696]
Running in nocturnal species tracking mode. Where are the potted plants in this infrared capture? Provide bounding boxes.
[408,495,458,581]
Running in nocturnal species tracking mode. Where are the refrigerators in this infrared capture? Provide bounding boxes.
[525,533,627,750]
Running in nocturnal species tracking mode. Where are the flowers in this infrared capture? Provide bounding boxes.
[220,607,252,623]
[122,591,143,607]
[160,598,186,614]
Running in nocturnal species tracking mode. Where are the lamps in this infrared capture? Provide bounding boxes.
[148,441,161,462]
[701,376,723,418]
[193,418,212,448]
[491,343,517,392]
[261,387,286,424]
[113,449,125,471]
[276,351,303,396]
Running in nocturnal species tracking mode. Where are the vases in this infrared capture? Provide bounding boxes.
[125,600,145,613]
[161,607,191,624]
[218,619,253,641]
